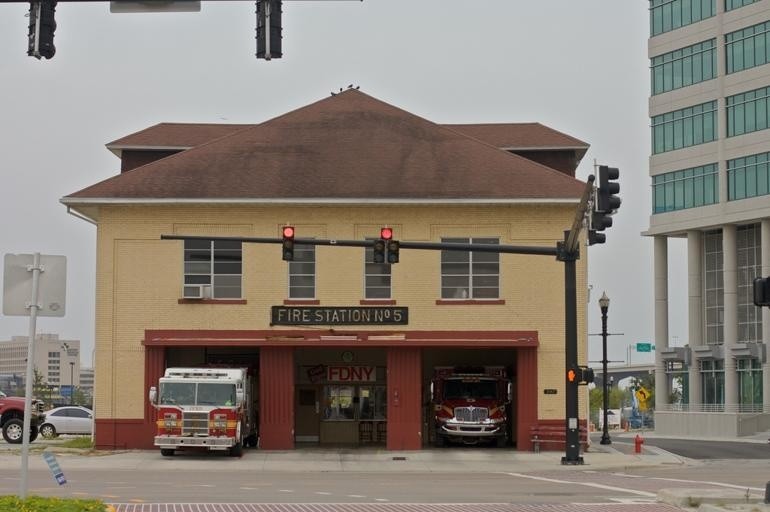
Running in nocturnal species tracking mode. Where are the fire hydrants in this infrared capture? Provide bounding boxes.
[635,434,644,455]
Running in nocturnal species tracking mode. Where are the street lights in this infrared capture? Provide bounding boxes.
[68,362,75,405]
[594,290,614,445]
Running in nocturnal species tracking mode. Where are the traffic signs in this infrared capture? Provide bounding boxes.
[637,343,652,352]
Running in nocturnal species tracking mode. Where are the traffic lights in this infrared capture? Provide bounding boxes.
[282,226,294,260]
[39,0,58,58]
[381,227,393,239]
[586,159,623,246]
[373,239,385,264]
[388,240,399,263]
[567,368,582,384]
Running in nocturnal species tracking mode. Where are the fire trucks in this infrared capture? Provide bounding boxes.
[148,363,259,459]
[428,363,514,449]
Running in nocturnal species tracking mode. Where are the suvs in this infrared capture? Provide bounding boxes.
[0,389,45,444]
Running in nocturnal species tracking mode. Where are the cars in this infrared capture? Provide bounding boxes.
[37,403,94,438]
